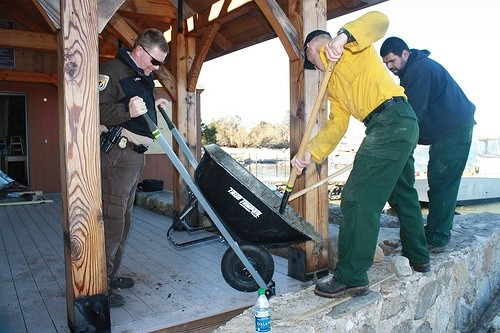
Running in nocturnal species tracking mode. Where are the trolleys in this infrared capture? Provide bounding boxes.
[133,97,322,293]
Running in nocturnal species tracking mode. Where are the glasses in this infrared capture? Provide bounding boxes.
[138,44,165,66]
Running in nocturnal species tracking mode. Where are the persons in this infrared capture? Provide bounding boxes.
[378,36,477,256]
[98,27,170,310]
[289,10,432,299]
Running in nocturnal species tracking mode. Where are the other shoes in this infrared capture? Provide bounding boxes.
[428,244,446,255]
[107,290,125,306]
[108,277,135,289]
[409,262,431,273]
[315,277,370,299]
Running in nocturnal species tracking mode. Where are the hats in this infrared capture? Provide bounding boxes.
[303,30,332,70]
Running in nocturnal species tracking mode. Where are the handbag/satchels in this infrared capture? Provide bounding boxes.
[137,179,164,192]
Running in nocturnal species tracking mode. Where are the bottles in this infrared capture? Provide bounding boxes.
[254,288,272,333]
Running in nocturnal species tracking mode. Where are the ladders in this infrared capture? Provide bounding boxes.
[1,136,10,156]
[10,134,26,156]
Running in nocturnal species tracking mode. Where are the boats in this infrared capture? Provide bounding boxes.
[412,134,500,208]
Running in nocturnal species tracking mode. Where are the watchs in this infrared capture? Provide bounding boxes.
[337,26,353,45]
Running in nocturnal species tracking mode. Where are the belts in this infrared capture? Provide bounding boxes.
[362,97,406,125]
